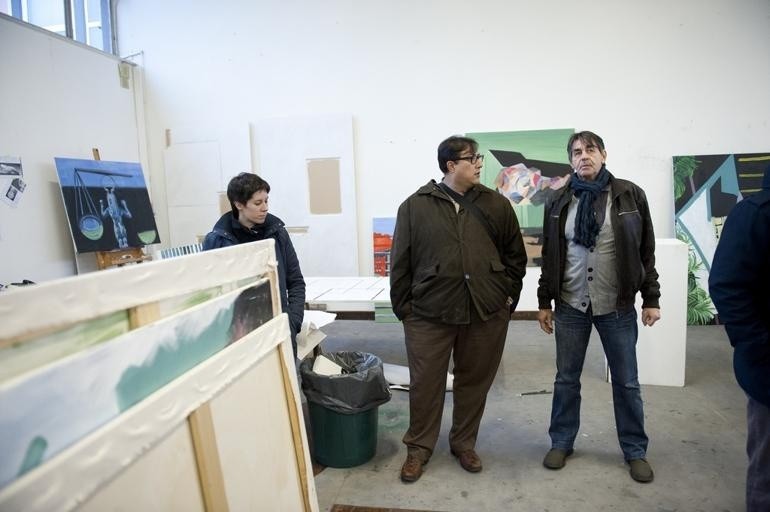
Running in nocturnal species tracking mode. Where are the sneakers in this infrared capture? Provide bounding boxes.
[544,446,573,469]
[627,458,654,482]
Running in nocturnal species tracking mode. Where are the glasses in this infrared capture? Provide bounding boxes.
[452,154,483,164]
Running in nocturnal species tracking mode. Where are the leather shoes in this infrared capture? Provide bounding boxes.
[451,448,482,473]
[401,455,428,482]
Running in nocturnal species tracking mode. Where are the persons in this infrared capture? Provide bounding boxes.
[389,136,528,483]
[708,161,769,512]
[202,172,305,364]
[536,130,661,483]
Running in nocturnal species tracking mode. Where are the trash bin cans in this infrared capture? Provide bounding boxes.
[299,350,382,469]
